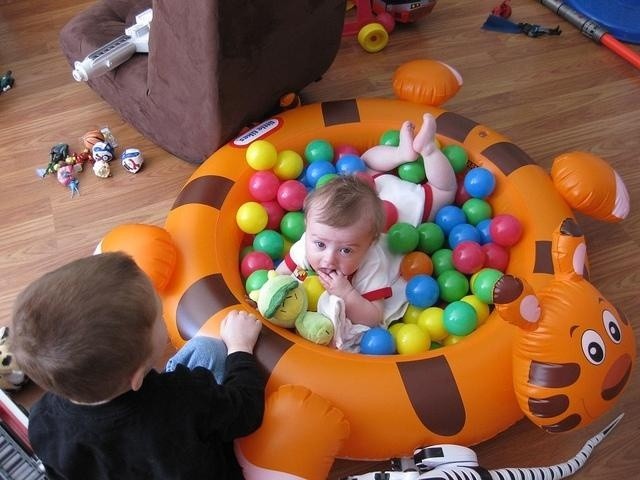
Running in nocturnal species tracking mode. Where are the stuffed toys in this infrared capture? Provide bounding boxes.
[250,270,333,347]
[0,326,29,391]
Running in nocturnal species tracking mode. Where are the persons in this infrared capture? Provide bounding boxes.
[276,112,458,328]
[6,248,270,480]
[518,21,562,38]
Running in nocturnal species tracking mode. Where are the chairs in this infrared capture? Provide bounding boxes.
[58,0,347,164]
